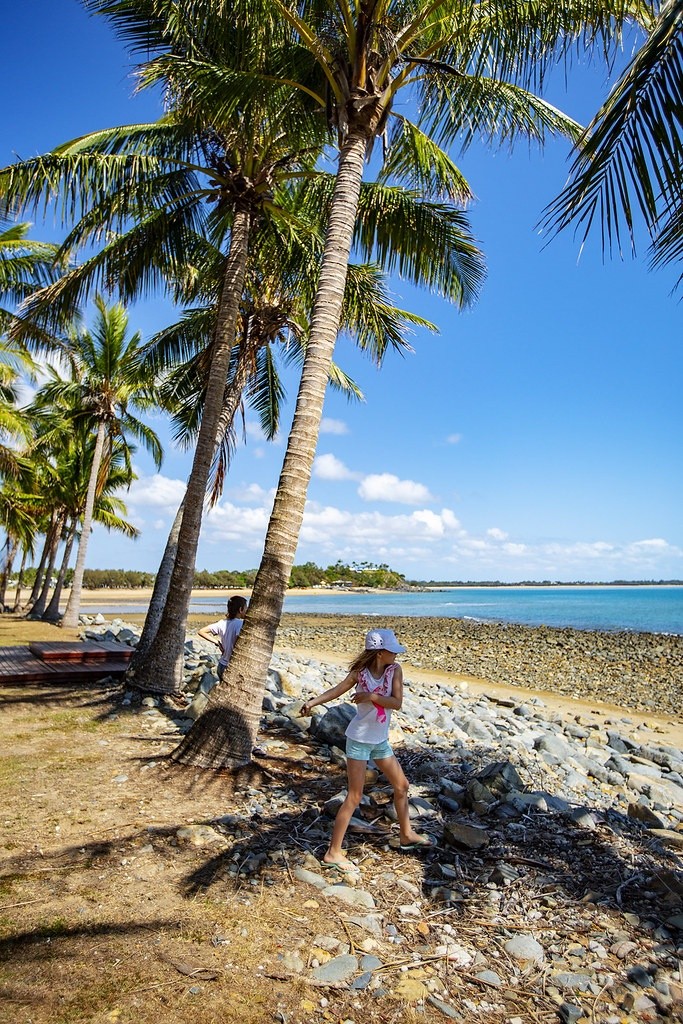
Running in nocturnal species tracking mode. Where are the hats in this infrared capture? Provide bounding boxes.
[365,628,408,653]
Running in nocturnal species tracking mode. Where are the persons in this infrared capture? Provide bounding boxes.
[298,628,437,874]
[196,595,248,686]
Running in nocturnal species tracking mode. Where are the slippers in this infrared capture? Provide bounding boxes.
[320,860,360,874]
[400,833,438,849]
